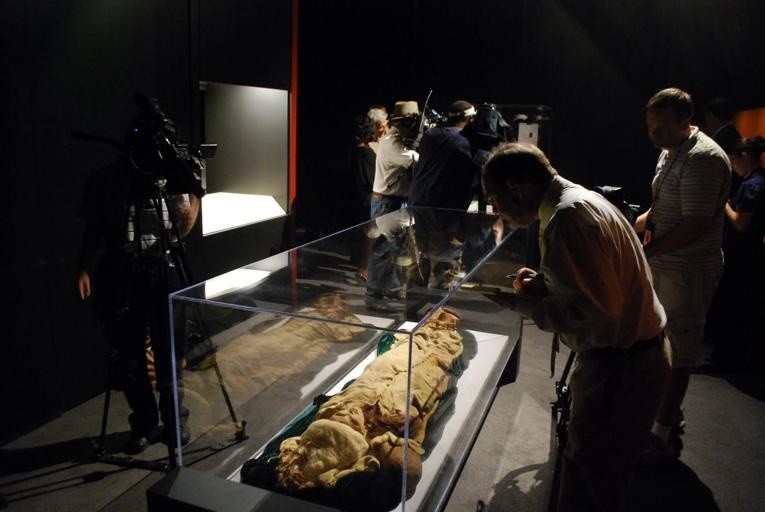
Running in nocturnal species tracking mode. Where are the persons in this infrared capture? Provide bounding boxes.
[353,98,520,314]
[695,122,748,375]
[701,131,765,374]
[631,87,729,467]
[475,139,677,511]
[52,95,207,452]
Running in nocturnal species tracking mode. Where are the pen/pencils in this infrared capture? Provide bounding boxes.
[505,273,542,279]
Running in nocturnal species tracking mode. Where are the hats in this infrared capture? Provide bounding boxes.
[391,101,420,120]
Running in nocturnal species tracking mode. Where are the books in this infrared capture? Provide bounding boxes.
[490,287,542,302]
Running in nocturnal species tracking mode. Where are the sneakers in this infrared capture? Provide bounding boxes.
[124,430,148,455]
[362,299,406,312]
[166,421,191,445]
[641,408,686,466]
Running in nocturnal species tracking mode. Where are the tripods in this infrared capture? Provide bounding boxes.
[87,196,246,472]
